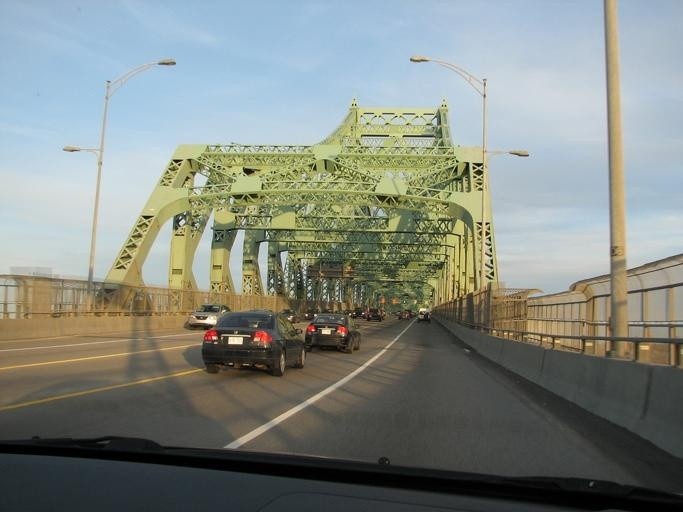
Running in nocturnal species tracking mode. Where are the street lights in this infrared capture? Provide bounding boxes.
[63,59,176,311]
[410,56,529,331]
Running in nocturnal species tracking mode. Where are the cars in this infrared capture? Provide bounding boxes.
[187,303,431,375]
